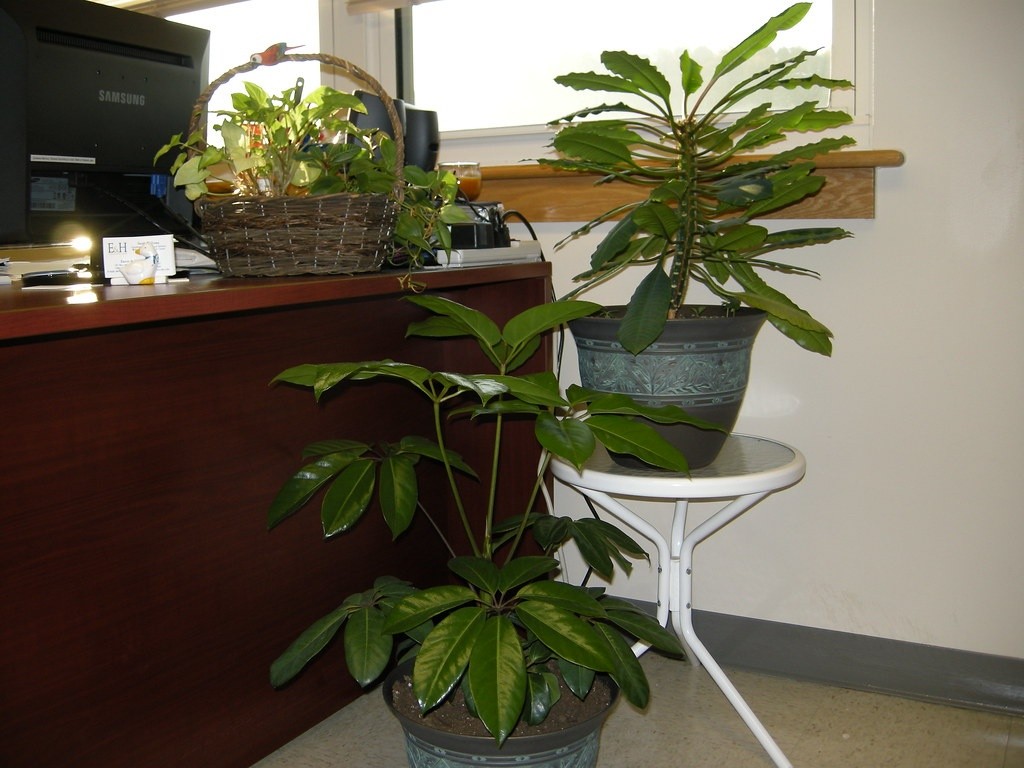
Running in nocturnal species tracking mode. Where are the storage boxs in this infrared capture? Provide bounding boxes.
[102,232,177,284]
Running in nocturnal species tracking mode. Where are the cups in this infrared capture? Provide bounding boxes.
[436,162,481,202]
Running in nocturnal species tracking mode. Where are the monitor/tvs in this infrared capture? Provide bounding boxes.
[0,0,201,283]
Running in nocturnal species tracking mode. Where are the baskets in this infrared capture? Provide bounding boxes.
[188,53,405,279]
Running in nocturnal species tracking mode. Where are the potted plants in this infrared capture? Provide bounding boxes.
[512,2,858,476]
[151,53,479,274]
[260,295,682,768]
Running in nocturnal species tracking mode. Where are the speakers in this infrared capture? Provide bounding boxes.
[343,91,438,194]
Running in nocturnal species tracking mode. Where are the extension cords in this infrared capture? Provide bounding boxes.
[436,240,541,267]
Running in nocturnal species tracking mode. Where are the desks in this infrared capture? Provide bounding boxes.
[547,433,809,768]
[0,264,560,768]
